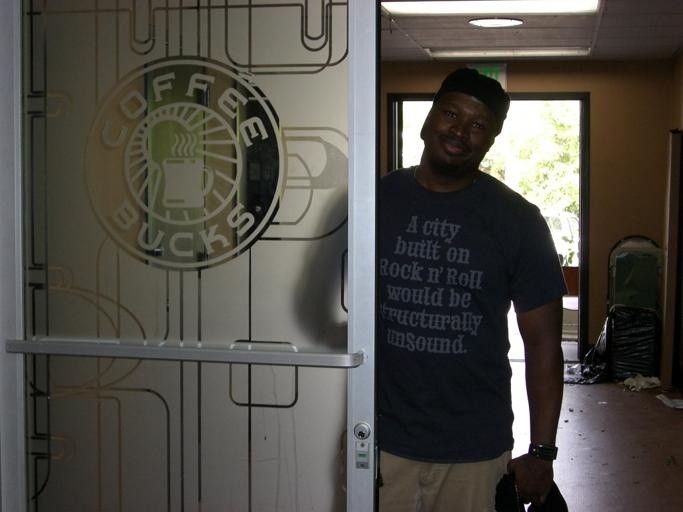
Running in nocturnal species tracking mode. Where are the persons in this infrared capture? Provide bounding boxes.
[289,67,567,511]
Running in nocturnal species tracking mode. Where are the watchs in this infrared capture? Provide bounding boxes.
[528,442,558,460]
